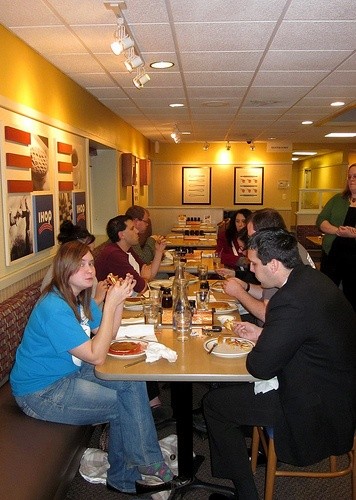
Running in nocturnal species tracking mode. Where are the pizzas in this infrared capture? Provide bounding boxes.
[150,234,160,241]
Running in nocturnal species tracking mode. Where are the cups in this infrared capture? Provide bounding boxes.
[196,292,209,310]
[174,310,192,341]
[173,252,180,268]
[212,253,221,270]
[198,264,208,283]
[142,290,162,329]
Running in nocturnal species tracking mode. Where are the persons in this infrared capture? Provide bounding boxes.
[202,227,356,500]
[91,215,167,408]
[316,162,356,309]
[10,240,205,500]
[40,226,108,312]
[220,209,316,328]
[126,205,161,265]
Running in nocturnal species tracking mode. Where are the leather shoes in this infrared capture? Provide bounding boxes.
[135,475,191,497]
[106,482,136,496]
[248,447,267,467]
[208,493,236,500]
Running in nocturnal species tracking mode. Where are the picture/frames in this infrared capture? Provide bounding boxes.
[233,167,264,205]
[182,167,211,205]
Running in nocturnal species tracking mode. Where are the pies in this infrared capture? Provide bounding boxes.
[107,273,122,286]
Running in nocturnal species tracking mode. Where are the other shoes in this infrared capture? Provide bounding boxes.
[151,403,173,424]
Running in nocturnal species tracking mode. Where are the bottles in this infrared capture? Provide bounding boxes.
[162,288,173,308]
[180,253,186,263]
[172,263,189,312]
[200,281,209,300]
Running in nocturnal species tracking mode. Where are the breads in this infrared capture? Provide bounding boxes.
[208,302,230,310]
[109,341,141,355]
[124,297,151,305]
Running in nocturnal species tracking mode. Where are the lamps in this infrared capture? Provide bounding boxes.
[132,67,151,90]
[201,140,256,151]
[111,18,134,56]
[170,126,183,145]
[124,46,144,73]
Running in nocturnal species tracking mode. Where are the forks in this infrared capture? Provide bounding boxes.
[208,344,217,355]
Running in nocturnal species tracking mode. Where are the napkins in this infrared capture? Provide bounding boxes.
[253,376,279,395]
[115,323,158,343]
[145,341,178,363]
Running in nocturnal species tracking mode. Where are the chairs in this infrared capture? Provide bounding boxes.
[251,425,356,500]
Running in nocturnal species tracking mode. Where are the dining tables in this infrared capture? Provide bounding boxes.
[154,249,225,273]
[162,221,217,247]
[94,280,265,500]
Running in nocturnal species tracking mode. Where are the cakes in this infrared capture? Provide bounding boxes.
[216,335,251,349]
[223,319,233,330]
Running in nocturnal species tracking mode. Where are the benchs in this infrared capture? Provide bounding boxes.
[292,225,326,259]
[0,277,93,500]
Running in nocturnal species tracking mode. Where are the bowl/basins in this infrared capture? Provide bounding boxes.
[218,316,234,324]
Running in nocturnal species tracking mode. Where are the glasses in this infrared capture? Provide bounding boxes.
[239,245,247,251]
[138,217,150,226]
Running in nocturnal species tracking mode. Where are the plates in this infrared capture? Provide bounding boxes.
[205,301,238,314]
[187,295,216,309]
[209,283,224,292]
[107,339,149,359]
[169,276,199,284]
[143,290,172,299]
[203,337,255,358]
[148,280,172,290]
[123,304,149,311]
[159,260,173,266]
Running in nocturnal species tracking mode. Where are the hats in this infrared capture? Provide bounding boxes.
[58,220,95,245]
